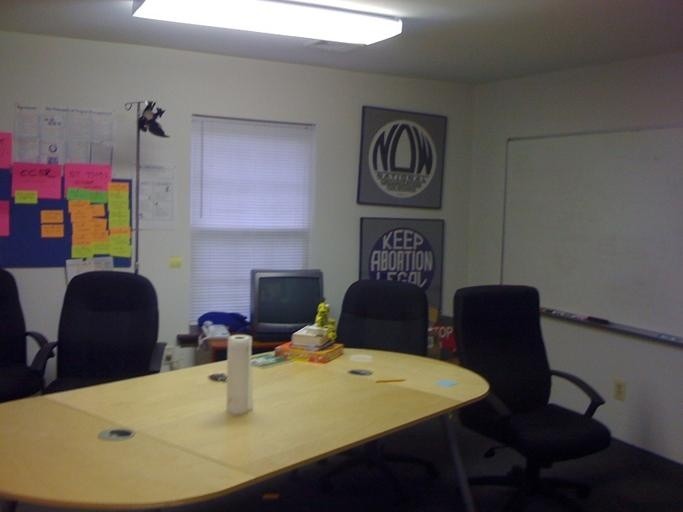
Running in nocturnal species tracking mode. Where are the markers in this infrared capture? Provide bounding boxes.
[540,308,609,326]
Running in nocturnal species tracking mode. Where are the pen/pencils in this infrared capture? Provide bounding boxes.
[376,379,405,383]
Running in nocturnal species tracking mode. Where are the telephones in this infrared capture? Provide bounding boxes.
[202,320,231,341]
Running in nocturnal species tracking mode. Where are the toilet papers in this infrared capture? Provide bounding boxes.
[226,334,254,414]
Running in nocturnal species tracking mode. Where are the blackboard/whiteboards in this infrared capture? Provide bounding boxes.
[500,119,683,347]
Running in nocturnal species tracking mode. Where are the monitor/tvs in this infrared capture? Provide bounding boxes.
[250,269,323,342]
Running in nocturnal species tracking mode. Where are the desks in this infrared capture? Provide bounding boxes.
[0,347,489,511]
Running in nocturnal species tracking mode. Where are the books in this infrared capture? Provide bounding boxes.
[274,323,343,364]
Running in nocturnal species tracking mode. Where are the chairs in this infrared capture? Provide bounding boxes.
[333,278,429,358]
[453,285,613,510]
[0,269,168,404]
